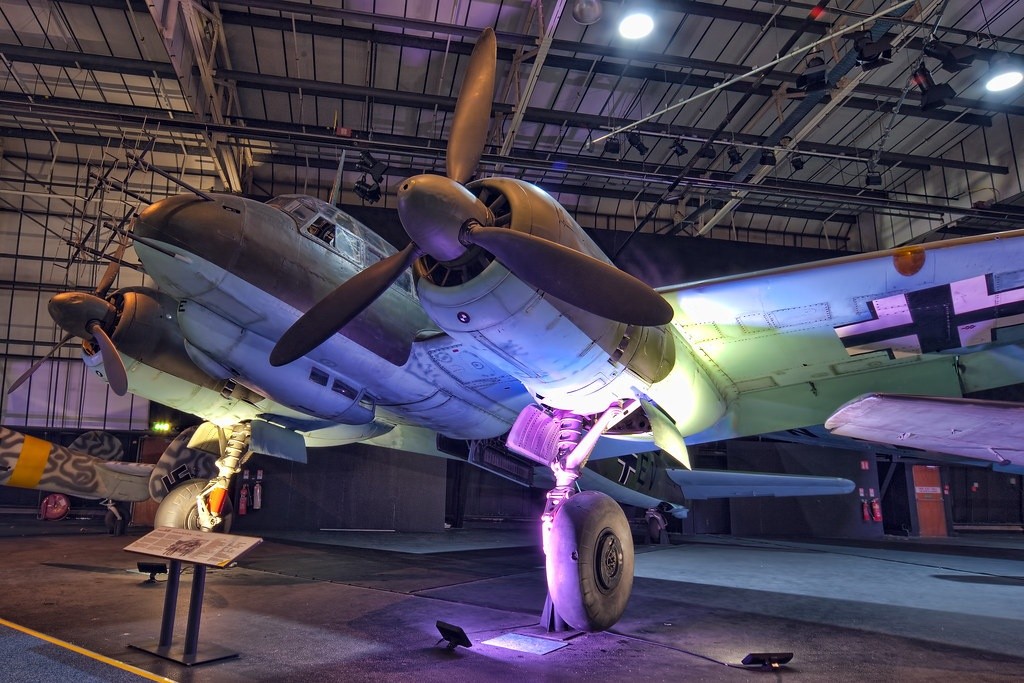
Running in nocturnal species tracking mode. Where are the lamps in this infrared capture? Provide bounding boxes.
[137,562,167,579]
[854,32,899,72]
[914,62,956,115]
[604,141,620,154]
[672,142,688,156]
[760,156,776,166]
[796,57,835,95]
[697,148,716,159]
[865,176,882,185]
[356,152,387,184]
[791,158,804,171]
[924,40,976,73]
[727,149,743,165]
[979,0,1024,94]
[436,620,472,649]
[741,653,793,665]
[626,133,649,155]
[353,181,382,205]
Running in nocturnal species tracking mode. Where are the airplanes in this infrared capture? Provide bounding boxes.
[0,24,1024,633]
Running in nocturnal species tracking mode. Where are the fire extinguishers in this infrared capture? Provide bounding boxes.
[860,497,870,520]
[239,479,250,515]
[867,497,882,521]
